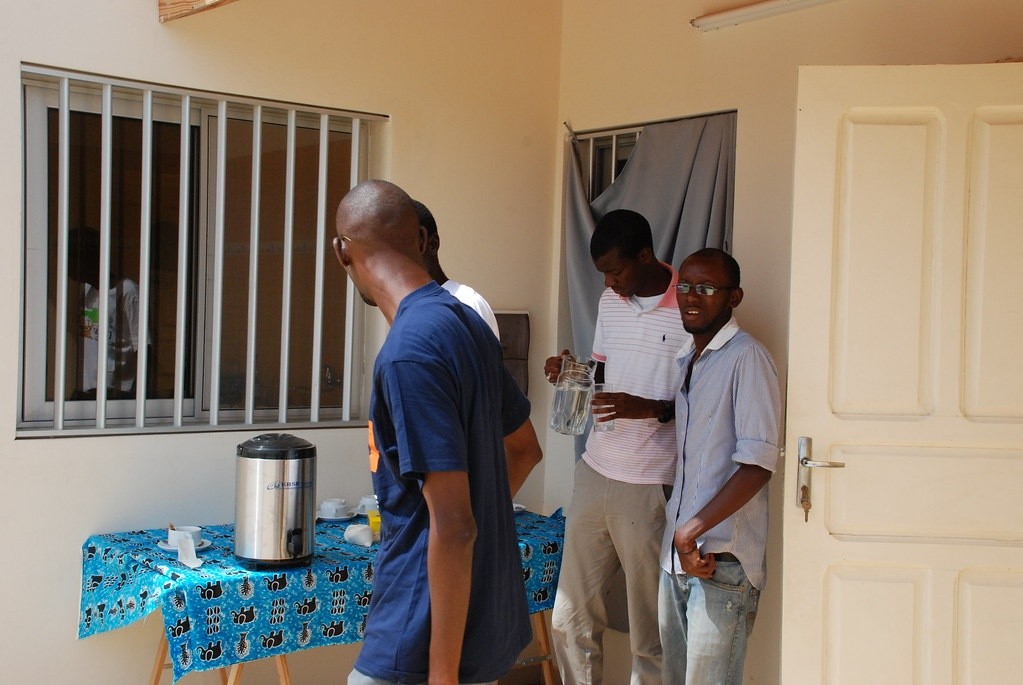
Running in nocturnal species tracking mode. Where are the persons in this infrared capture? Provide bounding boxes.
[67,225,152,401]
[333,179,542,685]
[660,248,782,685]
[543,210,692,685]
[412,198,500,342]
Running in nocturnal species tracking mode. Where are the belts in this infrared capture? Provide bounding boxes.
[713,550,739,562]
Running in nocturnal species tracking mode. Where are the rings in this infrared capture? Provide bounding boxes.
[547,372,551,380]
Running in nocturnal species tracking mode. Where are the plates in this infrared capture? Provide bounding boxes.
[316,511,357,521]
[351,508,379,517]
[157,538,211,553]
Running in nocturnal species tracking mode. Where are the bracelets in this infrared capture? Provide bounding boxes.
[678,541,697,555]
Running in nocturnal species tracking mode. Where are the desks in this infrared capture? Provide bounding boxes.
[83,509,565,685]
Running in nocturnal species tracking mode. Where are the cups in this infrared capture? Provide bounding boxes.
[357,495,377,512]
[592,384,615,432]
[320,498,348,516]
[168,526,202,548]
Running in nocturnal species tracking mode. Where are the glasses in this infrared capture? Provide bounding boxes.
[673,283,732,296]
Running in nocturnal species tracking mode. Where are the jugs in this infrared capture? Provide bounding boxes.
[549,355,595,436]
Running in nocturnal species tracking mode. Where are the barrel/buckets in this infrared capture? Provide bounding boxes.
[234,433,317,571]
